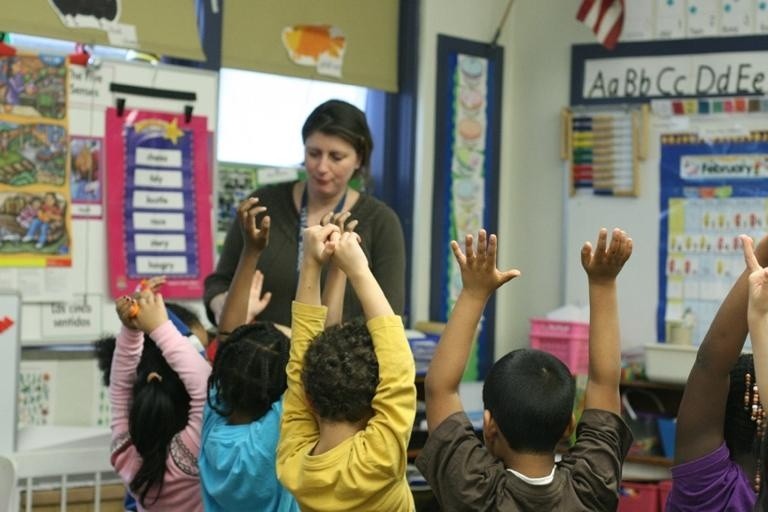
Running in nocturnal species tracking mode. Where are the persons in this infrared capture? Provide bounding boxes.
[85,285,210,512]
[204,99,405,335]
[737,236,767,508]
[194,196,360,511]
[661,232,768,512]
[272,221,418,512]
[93,268,274,512]
[416,226,634,512]
